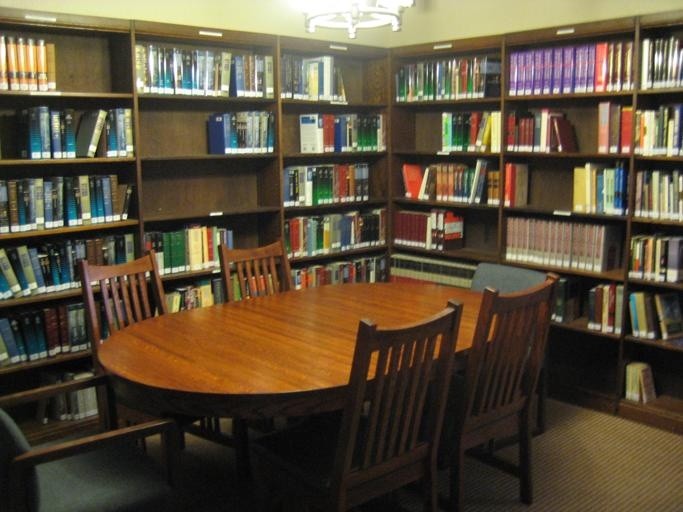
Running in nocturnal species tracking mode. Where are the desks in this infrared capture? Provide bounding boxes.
[96,284,495,512]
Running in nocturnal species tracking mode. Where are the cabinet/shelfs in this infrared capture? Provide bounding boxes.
[386,7,683,438]
[0,8,389,408]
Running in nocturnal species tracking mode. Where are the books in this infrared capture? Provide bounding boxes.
[586,280,624,335]
[505,216,621,273]
[280,52,349,101]
[153,268,280,318]
[597,100,633,155]
[207,109,276,154]
[289,253,387,290]
[627,233,683,284]
[18,106,135,160]
[0,296,128,370]
[144,224,234,277]
[503,162,530,208]
[634,102,683,157]
[640,34,683,91]
[134,41,275,99]
[283,160,370,208]
[0,34,58,93]
[508,40,634,96]
[551,276,581,323]
[298,112,384,154]
[284,206,387,260]
[0,173,133,234]
[400,159,500,206]
[503,106,578,154]
[624,360,658,405]
[572,159,630,216]
[628,290,683,341]
[441,110,501,154]
[0,232,135,300]
[388,252,479,288]
[34,370,100,425]
[393,54,500,103]
[634,169,683,222]
[392,207,465,251]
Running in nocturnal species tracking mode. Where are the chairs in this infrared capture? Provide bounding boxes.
[470,262,551,434]
[0,371,179,511]
[249,299,462,511]
[76,251,219,449]
[218,237,296,302]
[441,271,559,511]
